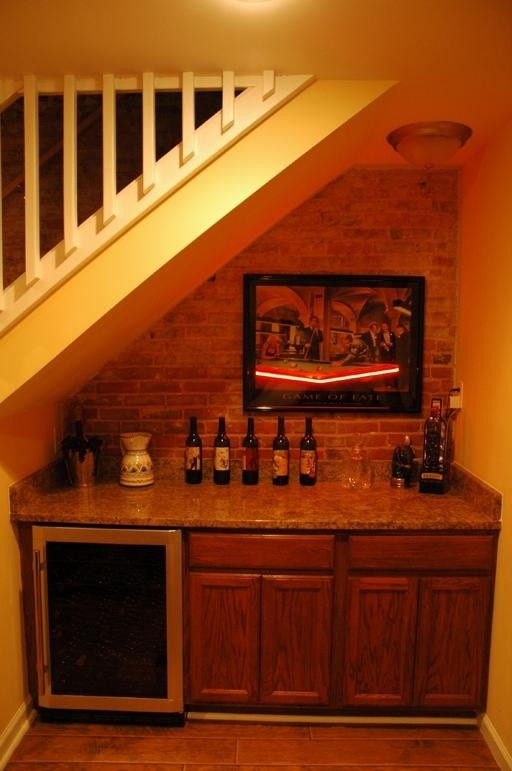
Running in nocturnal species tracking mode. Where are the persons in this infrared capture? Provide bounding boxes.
[261,314,410,388]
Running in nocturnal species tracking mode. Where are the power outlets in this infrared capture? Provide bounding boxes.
[453,379,463,408]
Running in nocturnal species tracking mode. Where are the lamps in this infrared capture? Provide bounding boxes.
[386,121,472,170]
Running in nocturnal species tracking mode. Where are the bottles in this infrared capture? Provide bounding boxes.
[71,407,89,447]
[212,417,231,486]
[298,416,319,485]
[422,398,445,494]
[388,433,416,489]
[348,444,371,488]
[241,418,259,486]
[184,417,203,484]
[117,431,154,487]
[271,415,290,486]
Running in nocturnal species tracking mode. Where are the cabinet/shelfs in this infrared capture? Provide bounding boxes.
[30,524,186,714]
[344,535,495,709]
[188,533,335,708]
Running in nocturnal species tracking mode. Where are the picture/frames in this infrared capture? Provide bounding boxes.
[241,272,424,417]
